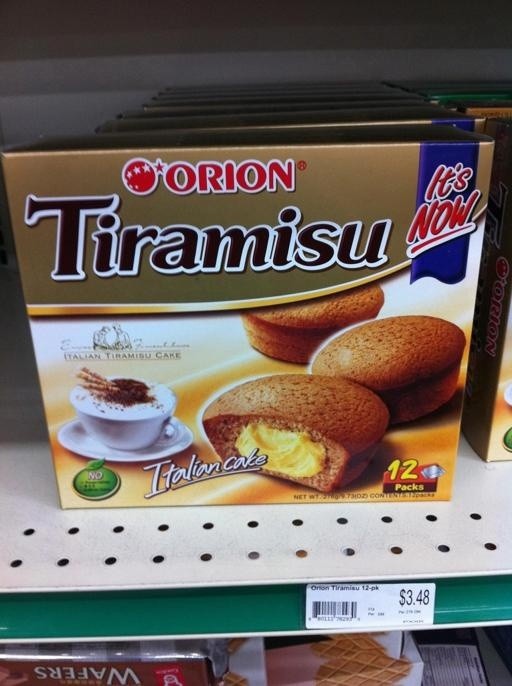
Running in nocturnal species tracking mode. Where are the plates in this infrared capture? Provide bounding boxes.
[52,419,196,462]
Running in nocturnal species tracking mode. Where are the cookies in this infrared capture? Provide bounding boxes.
[310,632,411,686]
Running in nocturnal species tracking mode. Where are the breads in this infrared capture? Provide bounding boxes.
[308,314,467,428]
[242,284,385,366]
[201,374,391,495]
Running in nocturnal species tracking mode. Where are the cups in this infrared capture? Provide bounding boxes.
[73,382,182,449]
